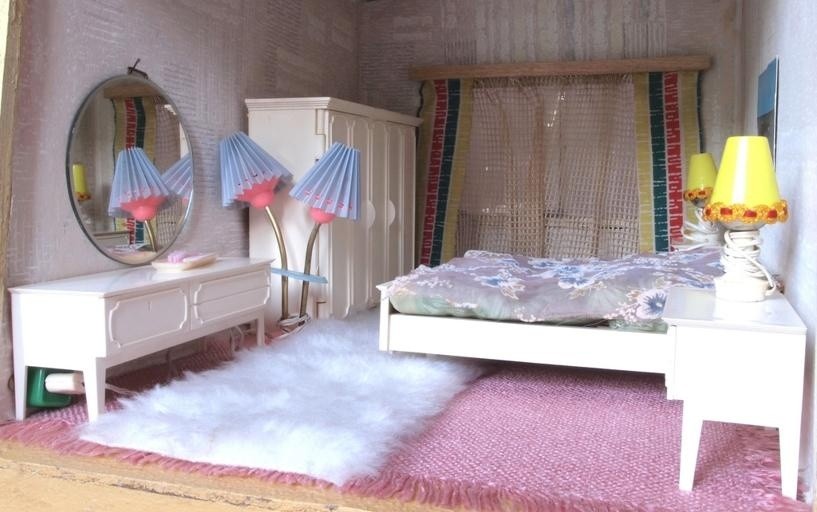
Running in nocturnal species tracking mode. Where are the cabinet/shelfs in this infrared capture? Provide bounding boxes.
[7,249,276,428]
[244,95,424,320]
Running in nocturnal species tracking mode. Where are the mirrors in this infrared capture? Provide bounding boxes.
[66,73,194,267]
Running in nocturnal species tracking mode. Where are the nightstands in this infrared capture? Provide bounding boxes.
[660,283,807,502]
[669,236,708,252]
[88,229,132,248]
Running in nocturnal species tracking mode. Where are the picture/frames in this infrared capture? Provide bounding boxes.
[756,55,779,171]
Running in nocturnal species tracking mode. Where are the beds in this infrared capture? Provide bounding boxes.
[376,248,786,373]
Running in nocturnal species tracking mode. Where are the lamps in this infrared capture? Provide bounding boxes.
[70,160,91,207]
[217,131,361,334]
[681,152,721,253]
[106,146,192,254]
[700,136,788,304]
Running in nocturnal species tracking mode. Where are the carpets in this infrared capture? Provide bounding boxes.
[1,310,812,512]
[74,315,492,486]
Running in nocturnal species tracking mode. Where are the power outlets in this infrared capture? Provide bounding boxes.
[27,367,74,410]
